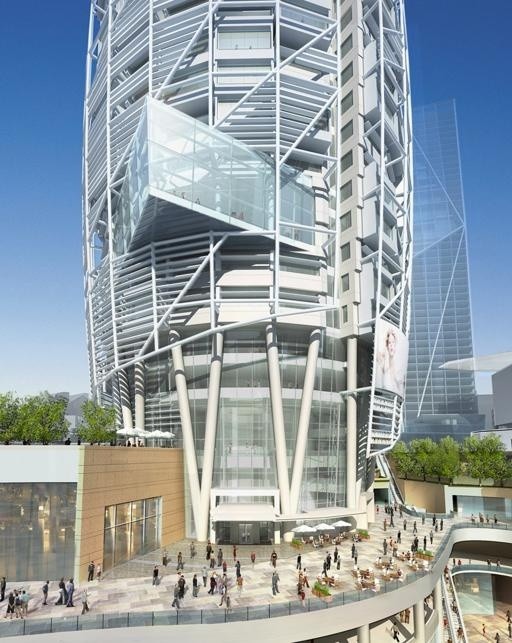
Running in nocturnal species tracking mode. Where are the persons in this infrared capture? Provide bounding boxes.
[0,558,104,618]
[65,437,72,444]
[151,500,511,643]
[376,326,405,396]
[78,437,81,444]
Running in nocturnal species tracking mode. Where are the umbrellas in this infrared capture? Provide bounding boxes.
[116,425,176,447]
